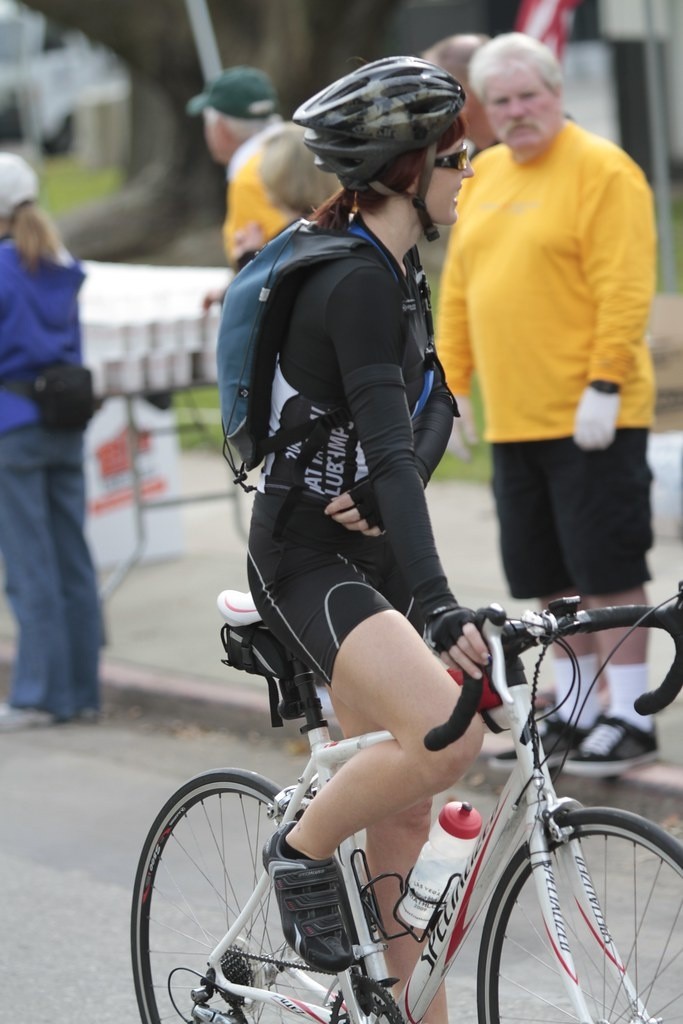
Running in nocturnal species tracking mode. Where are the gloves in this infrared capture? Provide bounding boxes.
[445,397,478,463]
[572,387,620,452]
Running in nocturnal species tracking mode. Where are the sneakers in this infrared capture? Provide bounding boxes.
[261,820,356,972]
[489,719,589,770]
[561,715,660,777]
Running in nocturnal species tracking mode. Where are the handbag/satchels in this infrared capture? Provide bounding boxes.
[1,365,95,432]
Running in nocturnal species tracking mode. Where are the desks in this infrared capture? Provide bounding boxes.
[75,264,253,608]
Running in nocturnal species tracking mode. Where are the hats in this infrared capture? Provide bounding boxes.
[184,65,277,119]
[0,150,38,220]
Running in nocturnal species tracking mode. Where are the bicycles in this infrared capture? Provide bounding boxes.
[128,575,683,1023]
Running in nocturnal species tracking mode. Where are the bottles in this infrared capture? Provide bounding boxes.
[397,801,483,930]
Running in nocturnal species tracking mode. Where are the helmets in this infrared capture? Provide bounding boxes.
[292,55,466,194]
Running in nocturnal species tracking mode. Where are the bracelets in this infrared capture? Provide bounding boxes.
[590,376,623,397]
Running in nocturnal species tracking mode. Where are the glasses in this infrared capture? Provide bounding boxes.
[434,139,467,172]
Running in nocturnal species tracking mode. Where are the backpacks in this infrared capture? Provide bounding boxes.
[214,216,411,473]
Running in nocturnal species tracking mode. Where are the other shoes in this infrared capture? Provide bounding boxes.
[0,702,54,733]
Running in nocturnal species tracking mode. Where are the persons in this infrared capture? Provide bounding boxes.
[427,29,673,777]
[200,123,342,300]
[0,151,114,735]
[184,62,303,264]
[237,42,497,1017]
